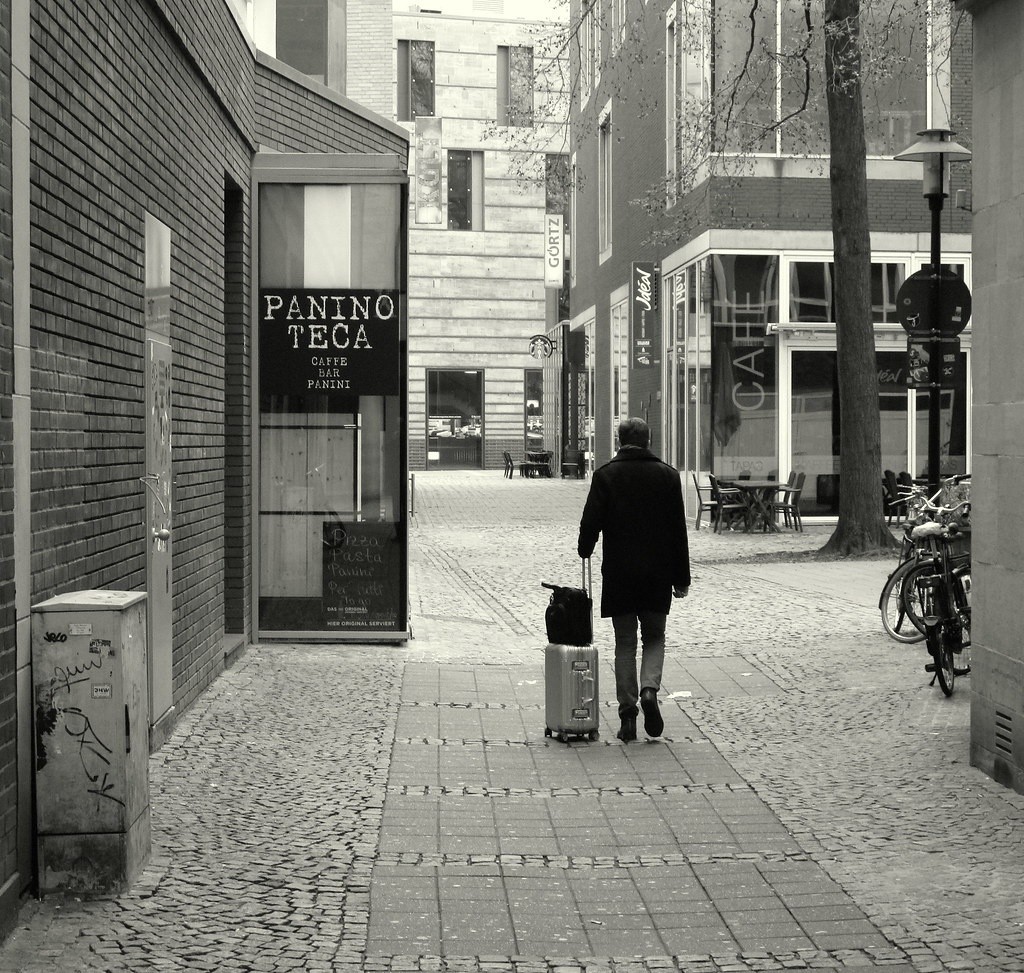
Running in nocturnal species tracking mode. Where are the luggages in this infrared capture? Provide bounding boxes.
[543,556,600,741]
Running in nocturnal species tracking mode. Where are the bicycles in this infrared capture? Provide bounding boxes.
[879,475,971,697]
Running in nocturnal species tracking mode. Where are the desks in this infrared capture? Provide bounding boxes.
[718,478,789,533]
[911,476,971,505]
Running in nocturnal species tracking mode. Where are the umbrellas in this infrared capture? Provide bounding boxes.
[712,340,744,483]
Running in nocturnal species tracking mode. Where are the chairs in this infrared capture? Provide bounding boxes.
[692,470,730,530]
[755,470,796,528]
[501,450,553,478]
[765,471,807,532]
[882,466,919,527]
[709,474,754,533]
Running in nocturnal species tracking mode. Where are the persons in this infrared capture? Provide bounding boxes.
[578,417,692,740]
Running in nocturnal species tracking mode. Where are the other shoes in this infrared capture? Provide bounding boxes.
[617,713,636,741]
[641,688,664,737]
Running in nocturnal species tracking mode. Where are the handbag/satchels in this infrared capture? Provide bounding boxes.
[541,583,593,647]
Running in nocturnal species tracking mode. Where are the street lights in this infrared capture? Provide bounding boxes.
[892,129,971,501]
